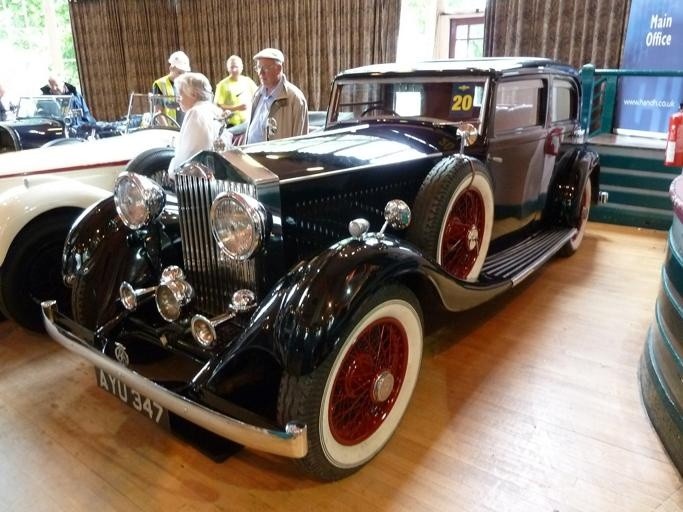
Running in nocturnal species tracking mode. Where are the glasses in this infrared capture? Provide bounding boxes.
[252,65,275,72]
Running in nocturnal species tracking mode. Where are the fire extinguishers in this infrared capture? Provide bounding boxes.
[664,101,683,166]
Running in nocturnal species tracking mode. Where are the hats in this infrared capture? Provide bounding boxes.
[253,48,285,63]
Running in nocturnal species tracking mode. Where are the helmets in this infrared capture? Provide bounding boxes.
[168,49,192,72]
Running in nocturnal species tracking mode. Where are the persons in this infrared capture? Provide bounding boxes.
[36,74,74,116]
[165,72,234,181]
[241,47,309,145]
[213,54,257,128]
[150,50,192,130]
